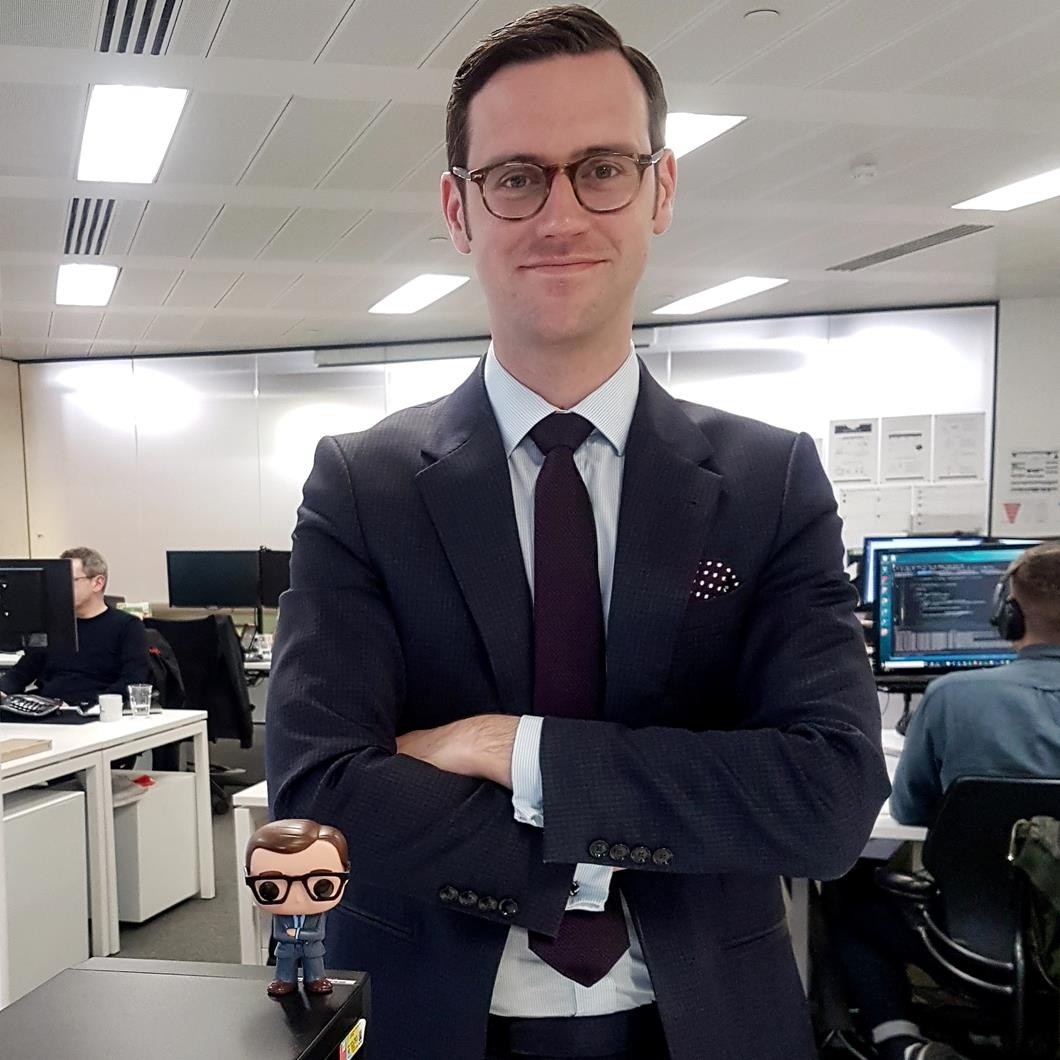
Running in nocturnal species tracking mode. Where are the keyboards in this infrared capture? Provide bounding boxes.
[0,694,62,717]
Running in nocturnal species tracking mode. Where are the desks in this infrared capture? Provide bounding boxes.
[1,707,214,1010]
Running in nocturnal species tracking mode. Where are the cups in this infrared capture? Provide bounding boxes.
[99,694,123,721]
[127,684,153,715]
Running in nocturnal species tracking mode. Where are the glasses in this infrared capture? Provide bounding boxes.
[452,148,666,220]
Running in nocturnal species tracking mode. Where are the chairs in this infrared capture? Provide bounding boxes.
[872,774,1060,1057]
[140,615,257,816]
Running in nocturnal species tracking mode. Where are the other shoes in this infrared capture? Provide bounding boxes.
[902,1034,966,1060]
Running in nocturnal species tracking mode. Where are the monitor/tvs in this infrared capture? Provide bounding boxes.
[0,559,78,698]
[164,548,291,607]
[862,534,1044,674]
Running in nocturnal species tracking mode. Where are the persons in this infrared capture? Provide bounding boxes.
[243,819,351,995]
[822,540,1060,1060]
[266,3,892,1060]
[0,547,149,787]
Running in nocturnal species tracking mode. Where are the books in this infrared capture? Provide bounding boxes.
[0,738,53,763]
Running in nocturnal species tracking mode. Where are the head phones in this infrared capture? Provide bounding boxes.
[991,559,1025,641]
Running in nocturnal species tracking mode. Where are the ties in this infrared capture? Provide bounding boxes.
[526,412,632,987]
